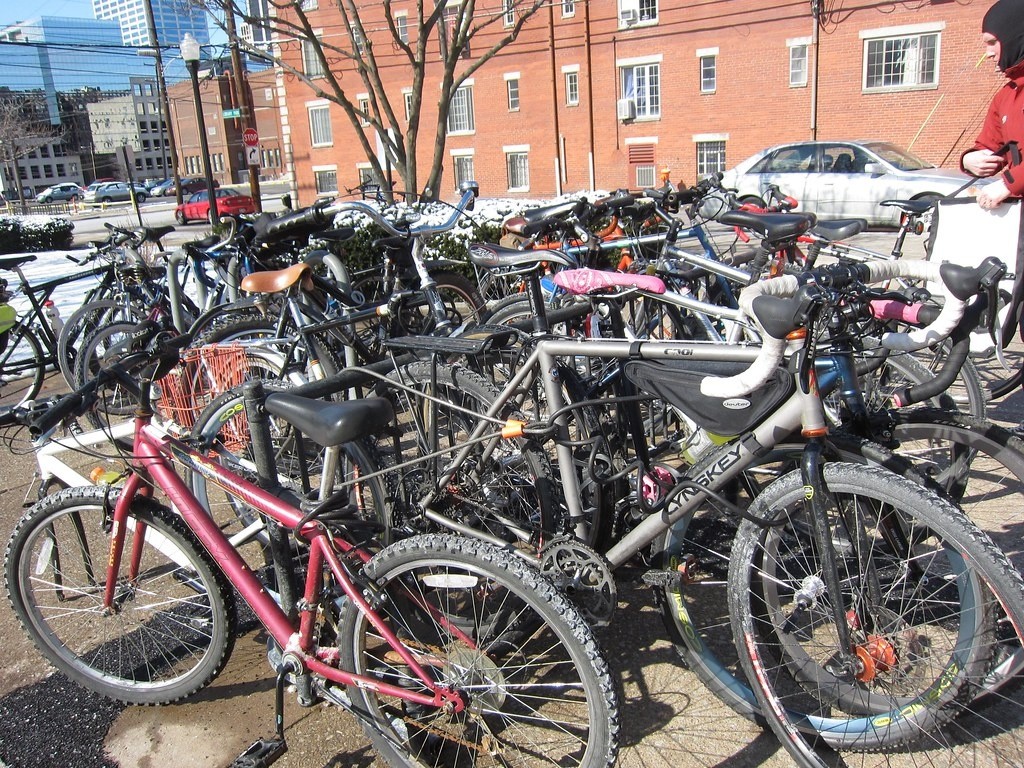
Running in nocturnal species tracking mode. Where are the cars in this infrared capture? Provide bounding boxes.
[174,187,255,226]
[34,176,220,210]
[697,140,1001,233]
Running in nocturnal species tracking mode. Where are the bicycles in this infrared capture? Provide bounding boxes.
[0,179,1020,768]
[2,300,625,768]
[341,243,1008,751]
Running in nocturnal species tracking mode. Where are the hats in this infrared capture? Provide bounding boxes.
[982,0,1024,71]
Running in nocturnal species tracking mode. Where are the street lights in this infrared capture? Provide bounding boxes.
[179,31,222,235]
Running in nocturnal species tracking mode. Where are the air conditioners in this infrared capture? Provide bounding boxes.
[620,9,639,22]
[617,99,635,120]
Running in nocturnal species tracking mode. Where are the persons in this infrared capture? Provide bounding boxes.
[960,0,1024,210]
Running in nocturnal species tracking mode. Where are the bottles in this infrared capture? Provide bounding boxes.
[89,466,127,490]
[45,300,64,341]
[682,428,740,465]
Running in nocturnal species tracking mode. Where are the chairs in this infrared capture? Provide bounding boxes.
[824,155,833,172]
[835,153,853,171]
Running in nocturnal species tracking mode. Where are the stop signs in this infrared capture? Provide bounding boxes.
[243,128,259,146]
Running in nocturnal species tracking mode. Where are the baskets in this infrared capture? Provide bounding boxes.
[155,347,249,456]
